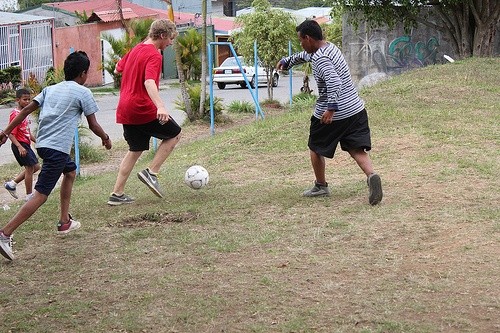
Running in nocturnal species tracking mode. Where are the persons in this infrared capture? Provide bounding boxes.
[275,21,383,206]
[0,51,112,260]
[4,89,40,199]
[108,19,182,205]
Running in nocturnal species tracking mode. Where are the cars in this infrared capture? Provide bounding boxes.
[212,56,280,89]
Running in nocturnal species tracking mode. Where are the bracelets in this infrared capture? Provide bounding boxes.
[3,132,8,137]
[105,134,109,140]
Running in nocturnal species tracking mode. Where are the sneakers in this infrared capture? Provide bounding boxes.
[0,228,16,262]
[301,182,330,197]
[138,167,164,198]
[367,173,383,205]
[57,214,81,232]
[108,192,135,205]
[4,181,20,200]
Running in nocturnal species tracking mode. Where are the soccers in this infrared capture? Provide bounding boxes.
[185,165,209,189]
